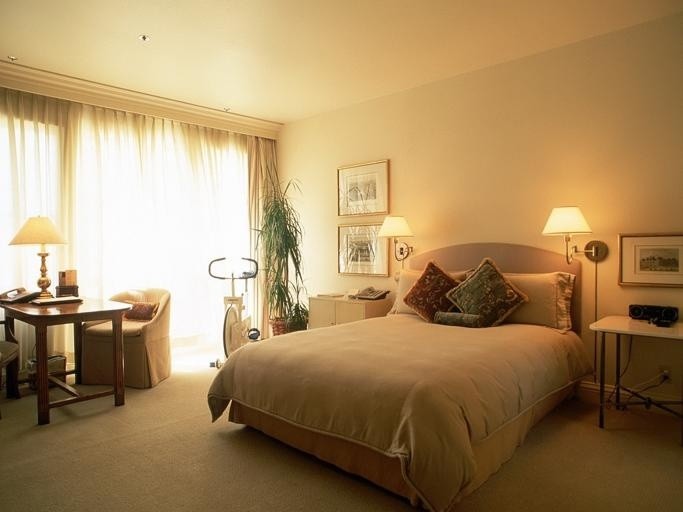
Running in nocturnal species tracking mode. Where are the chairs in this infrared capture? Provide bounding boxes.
[0,320,21,420]
[79,288,171,390]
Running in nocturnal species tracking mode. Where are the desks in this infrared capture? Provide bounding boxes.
[589,314,683,430]
[0,292,134,425]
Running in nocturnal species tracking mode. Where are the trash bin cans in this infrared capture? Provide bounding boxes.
[26,355,66,390]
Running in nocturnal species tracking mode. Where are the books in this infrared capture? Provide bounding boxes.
[30,295,83,305]
[316,292,345,298]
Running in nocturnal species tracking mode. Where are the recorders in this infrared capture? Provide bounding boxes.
[629,305,679,322]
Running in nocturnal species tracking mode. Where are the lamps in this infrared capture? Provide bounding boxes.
[377,215,415,261]
[8,216,68,300]
[541,207,609,265]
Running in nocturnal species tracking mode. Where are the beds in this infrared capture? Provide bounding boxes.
[205,242,598,512]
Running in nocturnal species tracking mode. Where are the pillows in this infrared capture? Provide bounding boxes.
[444,257,531,328]
[466,270,577,335]
[436,311,481,328]
[403,260,461,324]
[386,268,475,316]
[124,300,160,321]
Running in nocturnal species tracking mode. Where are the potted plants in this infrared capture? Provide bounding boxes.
[249,159,309,336]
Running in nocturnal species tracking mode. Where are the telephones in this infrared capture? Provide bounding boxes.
[0,287,41,303]
[358,287,390,300]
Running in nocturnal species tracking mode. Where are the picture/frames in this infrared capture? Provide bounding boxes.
[617,232,683,289]
[337,158,391,217]
[337,223,390,279]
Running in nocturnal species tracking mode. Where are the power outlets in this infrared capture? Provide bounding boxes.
[656,365,671,384]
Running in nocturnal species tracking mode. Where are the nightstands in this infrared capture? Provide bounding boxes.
[309,293,393,331]
